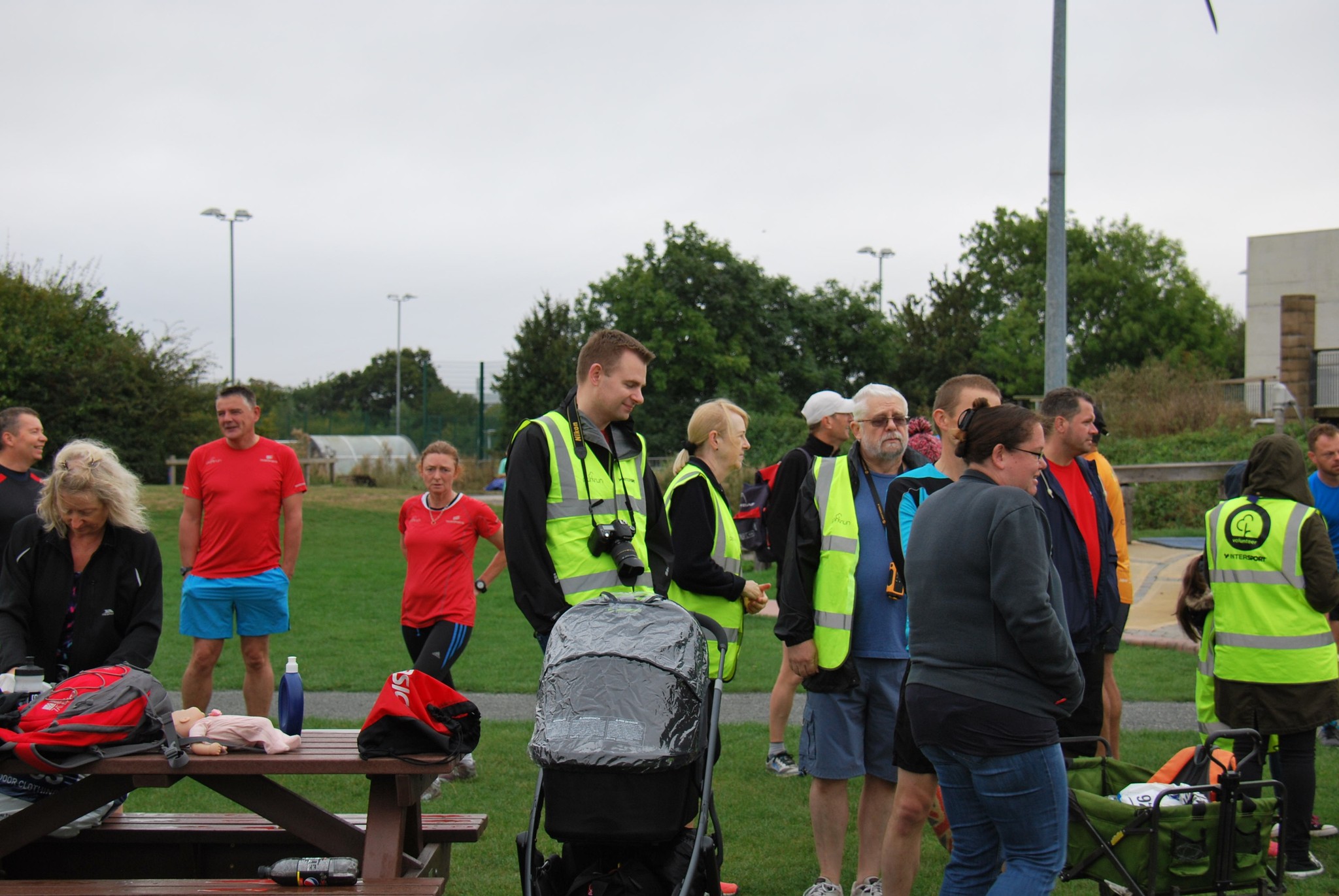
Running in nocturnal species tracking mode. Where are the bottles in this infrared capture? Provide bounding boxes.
[278,656,304,737]
[258,857,359,886]
[14,657,44,707]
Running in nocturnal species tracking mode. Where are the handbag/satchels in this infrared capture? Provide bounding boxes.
[359,669,480,758]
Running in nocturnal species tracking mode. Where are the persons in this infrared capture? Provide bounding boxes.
[502,329,660,654]
[178,386,307,717]
[1176,424,1339,876]
[398,441,508,803]
[664,398,773,690]
[0,406,51,538]
[0,438,164,670]
[761,373,1133,896]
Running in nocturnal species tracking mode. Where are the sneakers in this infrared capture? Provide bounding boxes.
[1284,856,1323,876]
[718,882,737,896]
[851,876,883,896]
[1318,727,1339,745]
[1309,815,1337,835]
[421,786,440,800]
[803,876,843,896]
[438,761,477,780]
[766,753,799,777]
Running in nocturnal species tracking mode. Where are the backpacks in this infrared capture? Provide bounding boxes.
[0,663,187,776]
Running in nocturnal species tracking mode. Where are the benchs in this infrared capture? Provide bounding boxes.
[0,811,488,843]
[0,877,448,896]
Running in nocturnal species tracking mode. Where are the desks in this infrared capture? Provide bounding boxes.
[0,727,463,893]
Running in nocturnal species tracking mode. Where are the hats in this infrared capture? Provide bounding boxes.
[801,391,855,425]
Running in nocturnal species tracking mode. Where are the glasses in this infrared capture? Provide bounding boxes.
[1009,446,1043,461]
[856,415,910,428]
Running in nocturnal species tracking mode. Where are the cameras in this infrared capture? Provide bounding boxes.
[587,517,645,588]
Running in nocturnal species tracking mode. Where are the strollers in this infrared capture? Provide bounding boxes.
[1050,727,1289,896]
[516,593,727,896]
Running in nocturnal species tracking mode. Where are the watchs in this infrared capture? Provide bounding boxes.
[475,580,487,594]
[179,566,193,576]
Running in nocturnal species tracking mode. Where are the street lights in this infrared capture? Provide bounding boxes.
[858,244,896,316]
[198,204,254,388]
[386,291,418,435]
